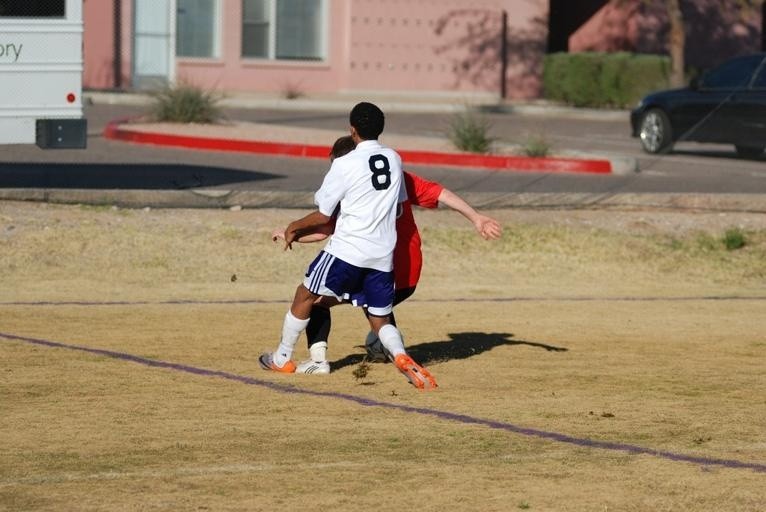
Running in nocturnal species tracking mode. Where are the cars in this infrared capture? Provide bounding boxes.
[632,54,766,156]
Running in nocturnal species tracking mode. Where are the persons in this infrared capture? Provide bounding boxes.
[272,135,505,375]
[258,102,439,390]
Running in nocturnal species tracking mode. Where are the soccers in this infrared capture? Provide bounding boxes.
[365,330,388,363]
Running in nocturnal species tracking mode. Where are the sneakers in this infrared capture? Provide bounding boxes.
[257,352,296,374]
[296,360,332,376]
[392,352,438,390]
